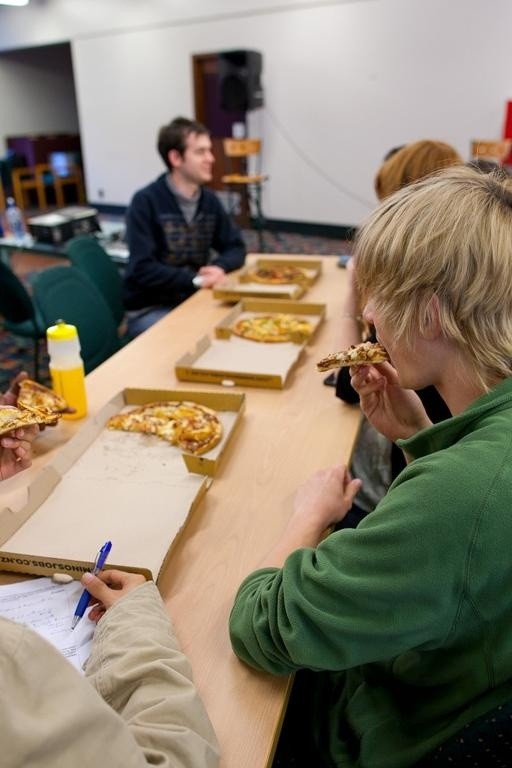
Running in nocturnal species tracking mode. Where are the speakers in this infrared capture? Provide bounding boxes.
[220,50,263,113]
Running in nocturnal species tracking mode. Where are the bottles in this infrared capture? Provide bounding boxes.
[47,318,88,421]
[6,196,25,241]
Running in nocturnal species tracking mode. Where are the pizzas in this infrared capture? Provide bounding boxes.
[108,400,224,455]
[233,313,312,343]
[317,342,390,372]
[0,380,69,436]
[247,263,307,284]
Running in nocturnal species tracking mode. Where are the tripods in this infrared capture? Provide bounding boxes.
[222,118,284,247]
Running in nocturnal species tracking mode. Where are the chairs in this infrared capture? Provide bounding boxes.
[27,258,125,380]
[221,139,273,252]
[0,257,50,379]
[65,232,144,320]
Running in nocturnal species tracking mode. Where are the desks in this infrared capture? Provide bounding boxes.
[0,251,371,766]
[0,219,131,269]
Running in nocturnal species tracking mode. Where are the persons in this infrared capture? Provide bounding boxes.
[0,569,225,767]
[0,370,77,482]
[1,260,53,394]
[227,171,511,768]
[332,140,464,534]
[120,117,245,341]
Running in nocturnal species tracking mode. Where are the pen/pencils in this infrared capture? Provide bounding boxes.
[70,540,114,631]
